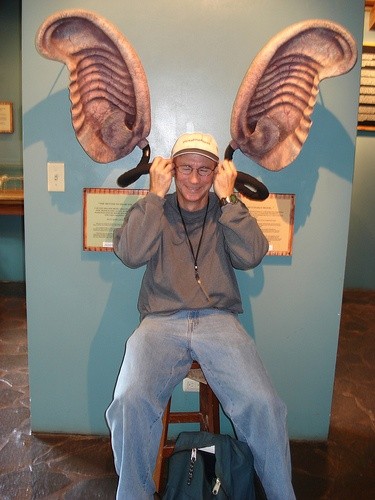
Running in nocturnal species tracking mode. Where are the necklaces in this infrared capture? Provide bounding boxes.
[176,194,210,281]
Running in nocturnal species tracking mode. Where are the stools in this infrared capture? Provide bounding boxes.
[153,362,222,492]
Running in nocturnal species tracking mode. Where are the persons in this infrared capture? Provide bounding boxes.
[106,132,297,500]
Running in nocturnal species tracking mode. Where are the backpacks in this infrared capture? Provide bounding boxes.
[160,430,258,500]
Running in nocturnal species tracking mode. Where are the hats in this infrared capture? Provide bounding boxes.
[169,133,220,163]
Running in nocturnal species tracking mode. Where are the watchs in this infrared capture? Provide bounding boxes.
[218,193,239,207]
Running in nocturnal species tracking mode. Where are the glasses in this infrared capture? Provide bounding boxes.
[176,165,214,176]
